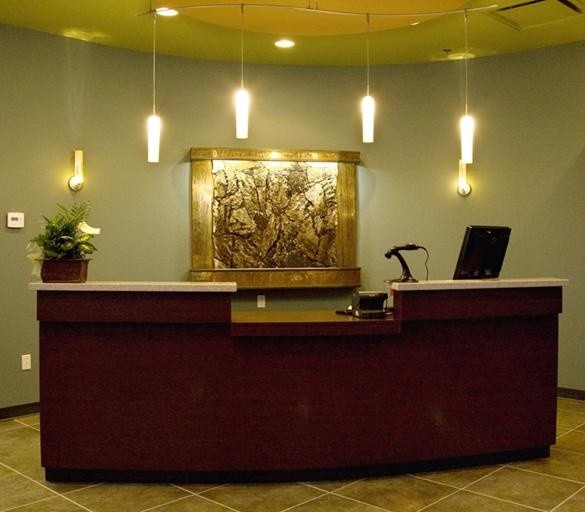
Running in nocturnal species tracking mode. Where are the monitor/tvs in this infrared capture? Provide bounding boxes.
[452,225,512,279]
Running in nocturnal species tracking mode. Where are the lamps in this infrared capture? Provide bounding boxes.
[69,149,86,192]
[456,160,479,199]
[135,4,478,168]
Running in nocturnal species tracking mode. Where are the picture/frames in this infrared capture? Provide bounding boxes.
[189,146,362,289]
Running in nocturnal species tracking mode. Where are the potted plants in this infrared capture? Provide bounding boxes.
[30,203,98,283]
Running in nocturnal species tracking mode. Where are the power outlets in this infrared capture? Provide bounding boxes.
[20,353,32,370]
